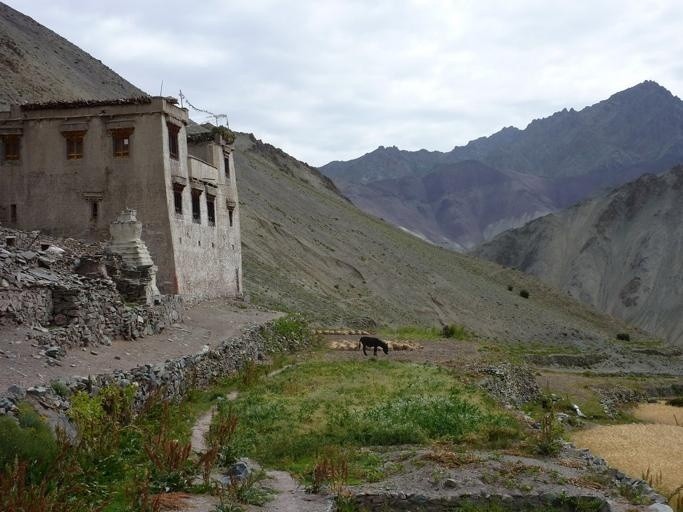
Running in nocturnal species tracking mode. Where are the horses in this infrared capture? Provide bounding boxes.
[358,336,389,358]
